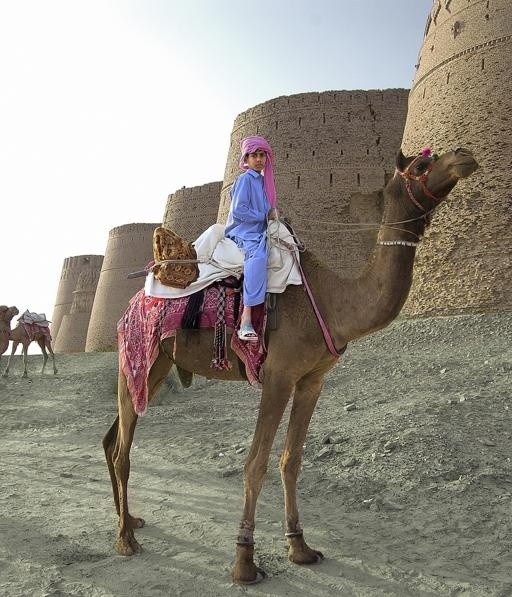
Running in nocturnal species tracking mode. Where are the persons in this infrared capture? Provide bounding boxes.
[224,135,278,341]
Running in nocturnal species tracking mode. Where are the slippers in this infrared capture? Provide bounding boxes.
[237,325,258,341]
